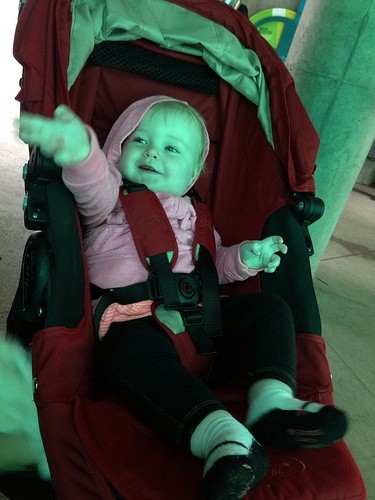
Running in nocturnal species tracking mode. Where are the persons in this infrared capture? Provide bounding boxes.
[15,94,348,500]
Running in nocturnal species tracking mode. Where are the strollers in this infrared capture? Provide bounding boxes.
[5,1,367,500]
